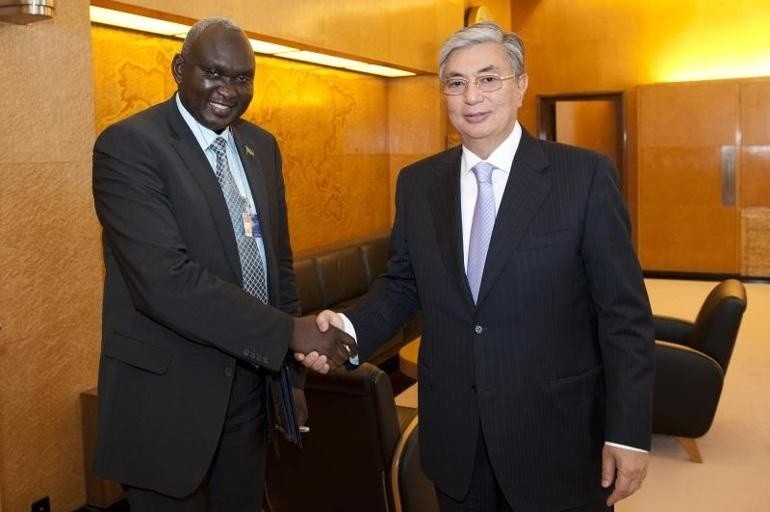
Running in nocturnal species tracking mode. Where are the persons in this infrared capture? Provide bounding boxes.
[90,17,359,512]
[295,21,657,512]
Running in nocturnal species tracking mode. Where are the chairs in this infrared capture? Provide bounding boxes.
[646,278,748,465]
[263,357,437,511]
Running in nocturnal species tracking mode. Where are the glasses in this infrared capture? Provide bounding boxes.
[442,72,512,94]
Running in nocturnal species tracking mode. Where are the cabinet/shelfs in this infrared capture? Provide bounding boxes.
[631,73,770,283]
[79,385,124,511]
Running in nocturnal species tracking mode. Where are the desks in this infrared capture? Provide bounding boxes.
[398,336,421,383]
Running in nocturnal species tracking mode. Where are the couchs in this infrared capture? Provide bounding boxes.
[292,230,392,318]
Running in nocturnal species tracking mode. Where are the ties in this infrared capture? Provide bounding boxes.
[209,136,270,307]
[466,162,497,305]
